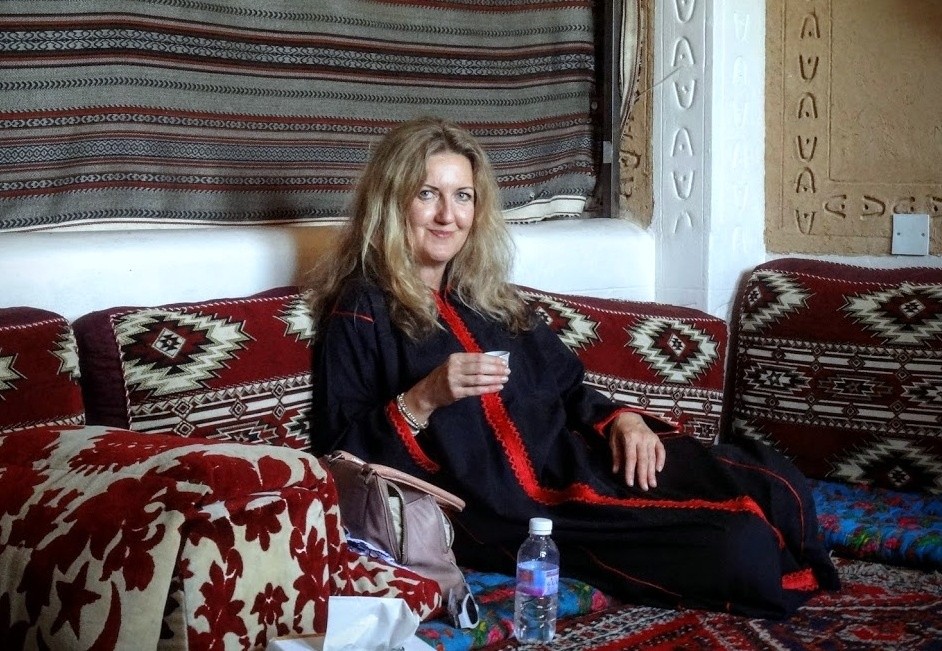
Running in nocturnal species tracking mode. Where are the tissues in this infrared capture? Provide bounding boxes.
[267,594,438,650]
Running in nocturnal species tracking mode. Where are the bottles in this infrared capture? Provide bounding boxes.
[514,517,560,645]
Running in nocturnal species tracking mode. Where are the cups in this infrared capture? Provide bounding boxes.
[484,351,510,368]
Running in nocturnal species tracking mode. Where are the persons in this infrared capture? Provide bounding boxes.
[298,116,844,623]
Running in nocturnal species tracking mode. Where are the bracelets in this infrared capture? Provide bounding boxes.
[396,392,429,437]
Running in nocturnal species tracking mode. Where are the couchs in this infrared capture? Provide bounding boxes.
[0,256,942,651]
